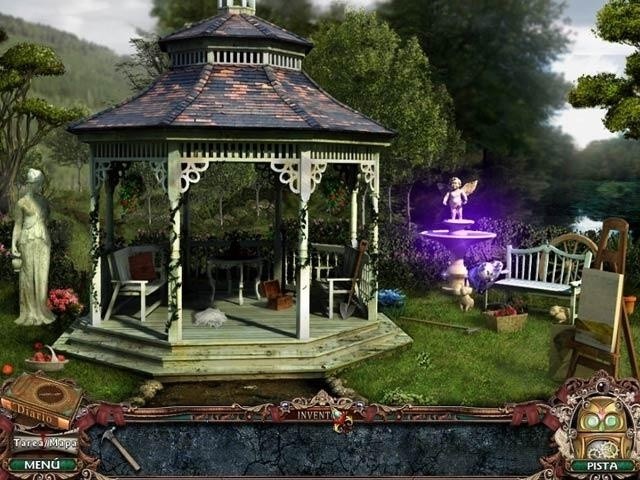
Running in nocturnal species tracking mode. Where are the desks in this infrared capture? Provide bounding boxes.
[206,254,271,308]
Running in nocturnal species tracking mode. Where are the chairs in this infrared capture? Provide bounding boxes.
[103,245,168,321]
[314,244,370,320]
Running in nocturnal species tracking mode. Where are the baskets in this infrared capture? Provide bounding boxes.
[483,307,529,334]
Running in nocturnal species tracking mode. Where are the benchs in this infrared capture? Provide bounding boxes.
[484,240,593,326]
